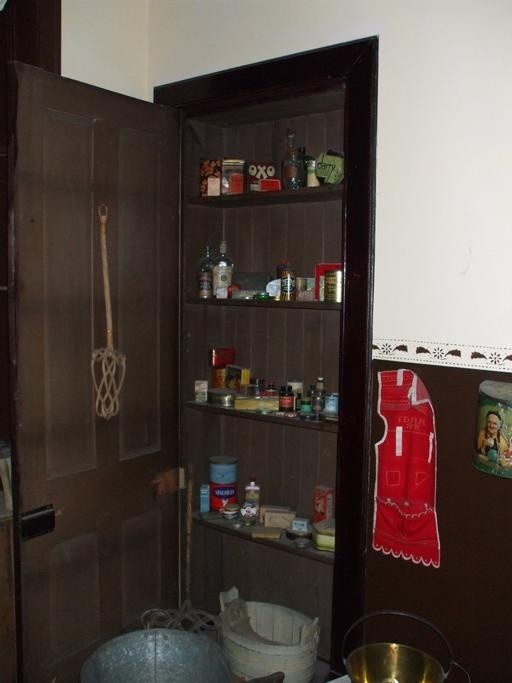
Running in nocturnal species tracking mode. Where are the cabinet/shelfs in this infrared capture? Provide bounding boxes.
[151,35,380,683]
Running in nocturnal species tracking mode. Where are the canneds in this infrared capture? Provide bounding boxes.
[280,267,296,303]
[323,270,342,302]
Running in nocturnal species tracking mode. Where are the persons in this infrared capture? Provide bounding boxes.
[479,409,509,468]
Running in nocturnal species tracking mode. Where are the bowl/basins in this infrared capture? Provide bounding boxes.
[256,291,275,300]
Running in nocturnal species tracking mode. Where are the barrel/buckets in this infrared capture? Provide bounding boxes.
[78,627,236,683]
[338,610,455,683]
[215,586,321,683]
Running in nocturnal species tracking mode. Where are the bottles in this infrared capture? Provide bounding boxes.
[276,257,296,302]
[198,238,232,299]
[244,480,260,500]
[282,130,307,188]
[251,372,324,420]
